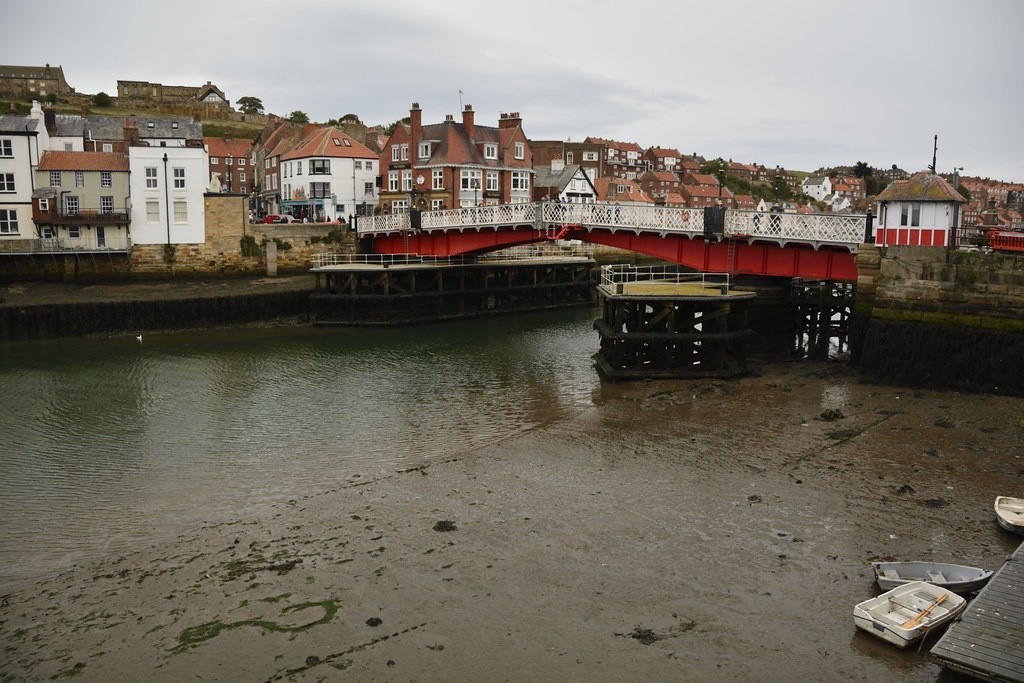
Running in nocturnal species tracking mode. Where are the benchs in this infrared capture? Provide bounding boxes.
[999,503,1024,513]
[866,609,902,627]
[913,592,955,615]
[79,211,97,219]
[889,596,937,618]
[926,570,947,583]
[883,570,900,579]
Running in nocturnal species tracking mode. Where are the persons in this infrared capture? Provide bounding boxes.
[326,215,330,222]
[770,201,784,234]
[587,197,621,224]
[551,196,576,220]
[337,213,352,223]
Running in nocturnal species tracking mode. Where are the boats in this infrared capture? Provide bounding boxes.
[871,561,995,595]
[852,581,968,650]
[994,496,1024,535]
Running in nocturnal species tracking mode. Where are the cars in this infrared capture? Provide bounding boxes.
[265,214,294,225]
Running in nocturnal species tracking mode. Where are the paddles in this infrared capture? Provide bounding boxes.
[899,593,948,629]
